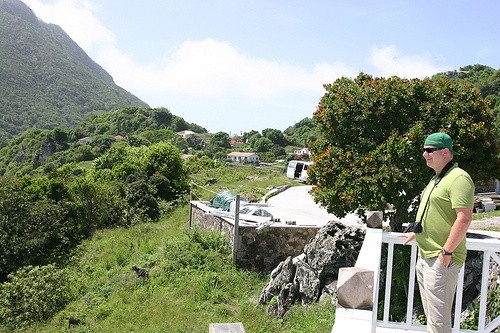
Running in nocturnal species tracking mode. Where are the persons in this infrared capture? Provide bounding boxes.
[395,130,476,333]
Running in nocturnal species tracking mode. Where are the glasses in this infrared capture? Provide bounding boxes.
[423,147,445,153]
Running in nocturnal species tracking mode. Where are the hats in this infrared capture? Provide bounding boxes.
[424,133,460,156]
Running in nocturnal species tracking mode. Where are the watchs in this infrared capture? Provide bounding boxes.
[442,248,454,256]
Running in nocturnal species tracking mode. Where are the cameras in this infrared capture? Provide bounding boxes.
[408,222,422,233]
